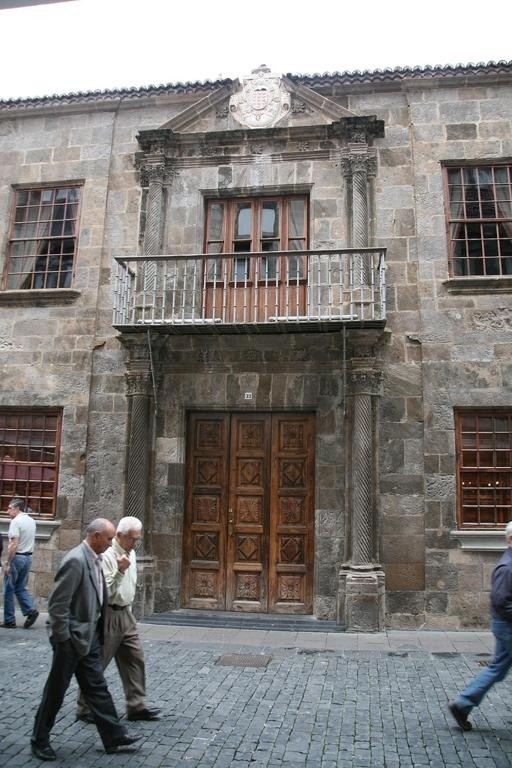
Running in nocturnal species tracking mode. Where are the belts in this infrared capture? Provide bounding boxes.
[15,551,34,556]
[107,603,126,610]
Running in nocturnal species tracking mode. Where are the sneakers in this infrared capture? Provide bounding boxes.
[0,620,17,628]
[447,702,473,732]
[25,610,38,628]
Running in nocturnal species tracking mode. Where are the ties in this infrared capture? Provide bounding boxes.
[96,559,102,587]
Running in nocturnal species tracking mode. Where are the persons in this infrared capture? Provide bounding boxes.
[31,518,142,760]
[0,499,39,628]
[448,522,512,731]
[76,516,161,725]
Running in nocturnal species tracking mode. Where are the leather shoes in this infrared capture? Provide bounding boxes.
[103,734,144,753]
[76,712,95,725]
[129,707,159,722]
[32,741,56,760]
